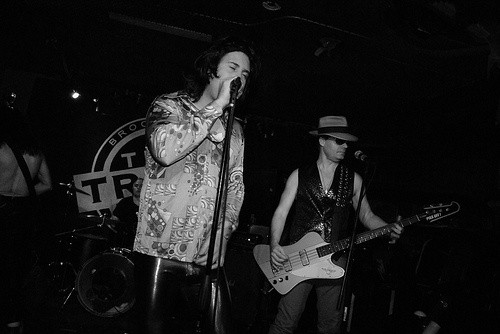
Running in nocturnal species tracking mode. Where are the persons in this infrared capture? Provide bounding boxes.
[131,41,255,334]
[270,116,403,334]
[0,110,53,327]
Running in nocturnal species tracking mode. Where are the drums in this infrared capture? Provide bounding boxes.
[74,252,140,318]
[69,235,114,267]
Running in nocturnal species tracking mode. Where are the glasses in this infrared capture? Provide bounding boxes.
[325,138,346,145]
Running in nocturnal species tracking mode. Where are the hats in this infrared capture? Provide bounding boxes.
[308,116,358,142]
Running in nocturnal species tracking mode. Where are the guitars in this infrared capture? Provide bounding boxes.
[253,200,460,295]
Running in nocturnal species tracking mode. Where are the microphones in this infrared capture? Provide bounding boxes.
[355,151,365,160]
[231,77,242,93]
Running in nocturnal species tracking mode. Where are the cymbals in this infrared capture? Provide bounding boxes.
[83,214,121,225]
